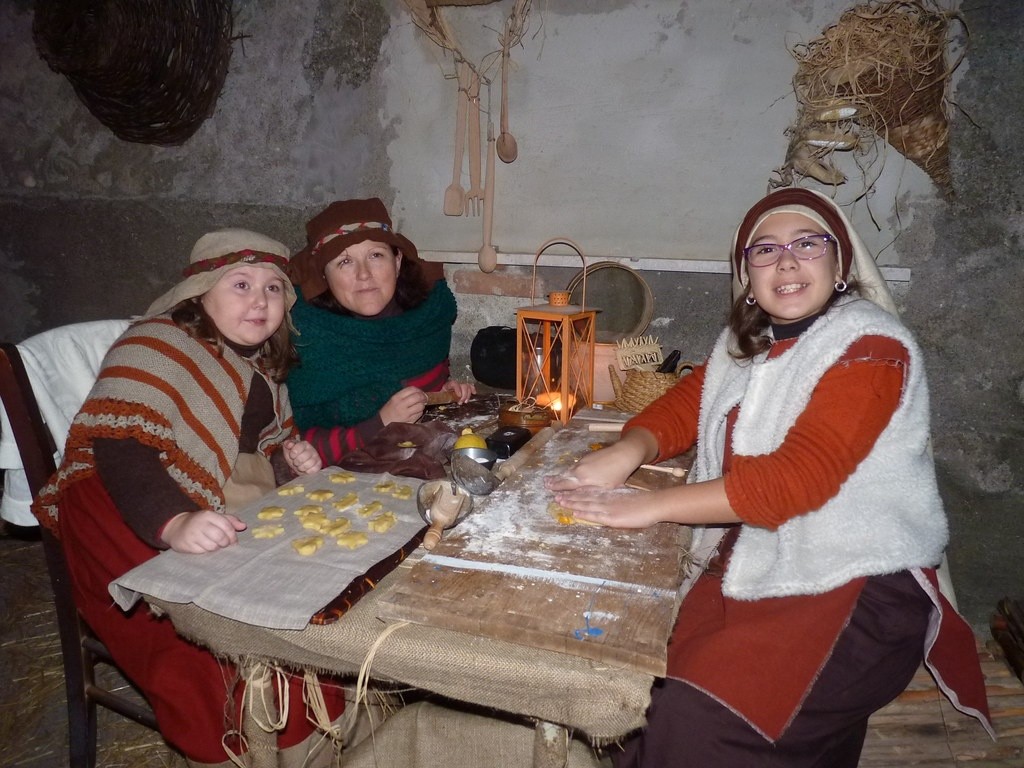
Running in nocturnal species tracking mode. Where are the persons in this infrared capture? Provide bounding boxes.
[30,228,346,768]
[548,186,997,768]
[284,197,477,470]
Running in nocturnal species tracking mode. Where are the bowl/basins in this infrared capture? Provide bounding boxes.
[454,447,497,470]
[497,404,552,435]
[417,479,474,527]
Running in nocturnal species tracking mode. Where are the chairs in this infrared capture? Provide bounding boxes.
[0,318,176,768]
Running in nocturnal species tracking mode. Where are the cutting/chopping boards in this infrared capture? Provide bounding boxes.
[378,419,697,678]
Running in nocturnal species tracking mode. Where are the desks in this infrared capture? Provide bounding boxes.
[106,435,700,768]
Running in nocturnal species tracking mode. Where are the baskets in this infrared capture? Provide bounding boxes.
[608,361,695,414]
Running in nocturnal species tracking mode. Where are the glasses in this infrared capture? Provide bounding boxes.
[741,235,836,268]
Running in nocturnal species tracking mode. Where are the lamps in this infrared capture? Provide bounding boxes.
[512,238,604,426]
